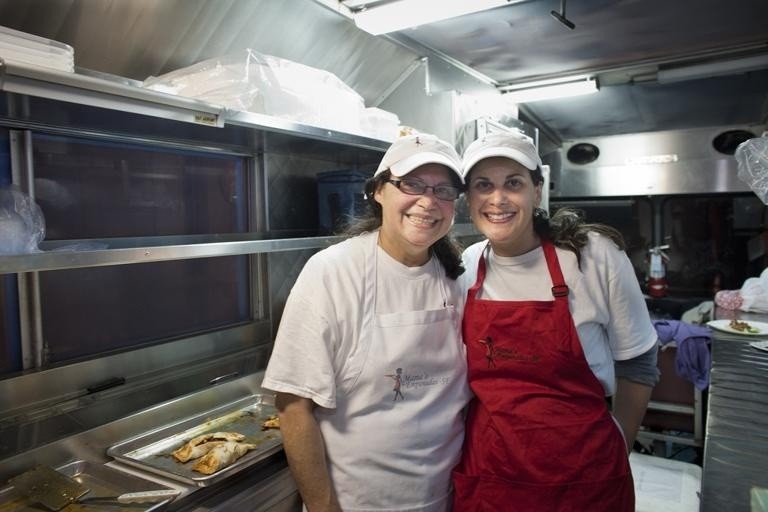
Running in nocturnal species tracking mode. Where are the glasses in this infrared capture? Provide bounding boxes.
[385,178,460,201]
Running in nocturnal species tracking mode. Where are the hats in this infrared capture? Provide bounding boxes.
[461,131,543,179]
[373,132,465,186]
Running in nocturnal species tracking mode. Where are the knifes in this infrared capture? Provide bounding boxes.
[49,376,125,406]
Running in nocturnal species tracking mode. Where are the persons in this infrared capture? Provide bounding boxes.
[259,132,474,512]
[448,131,661,512]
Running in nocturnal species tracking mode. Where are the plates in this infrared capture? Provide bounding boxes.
[706,319,767,337]
[749,340,767,352]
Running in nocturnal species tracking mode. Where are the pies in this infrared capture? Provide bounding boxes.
[168,430,255,477]
